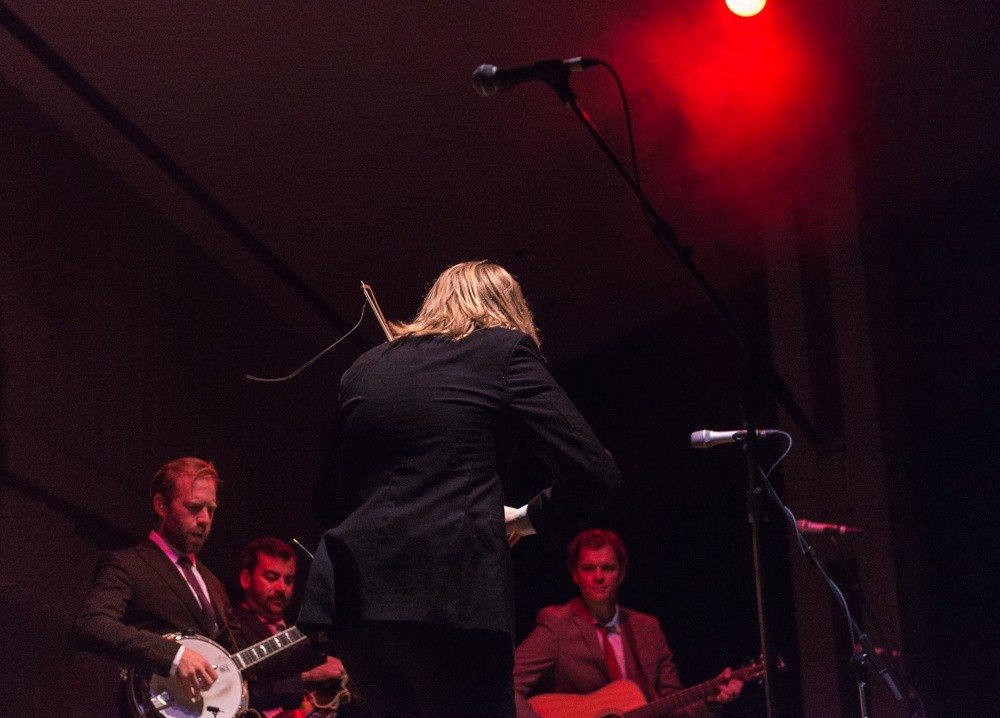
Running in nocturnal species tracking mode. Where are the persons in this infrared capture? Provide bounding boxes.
[295,261,621,718]
[513,528,743,718]
[72,457,346,718]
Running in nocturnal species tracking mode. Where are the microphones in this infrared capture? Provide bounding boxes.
[470,57,598,97]
[690,429,778,449]
[855,643,904,657]
[794,519,863,536]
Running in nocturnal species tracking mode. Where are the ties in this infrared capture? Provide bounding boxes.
[596,623,622,681]
[177,556,216,623]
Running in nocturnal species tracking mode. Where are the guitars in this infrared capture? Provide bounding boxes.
[125,624,309,718]
[526,648,784,718]
